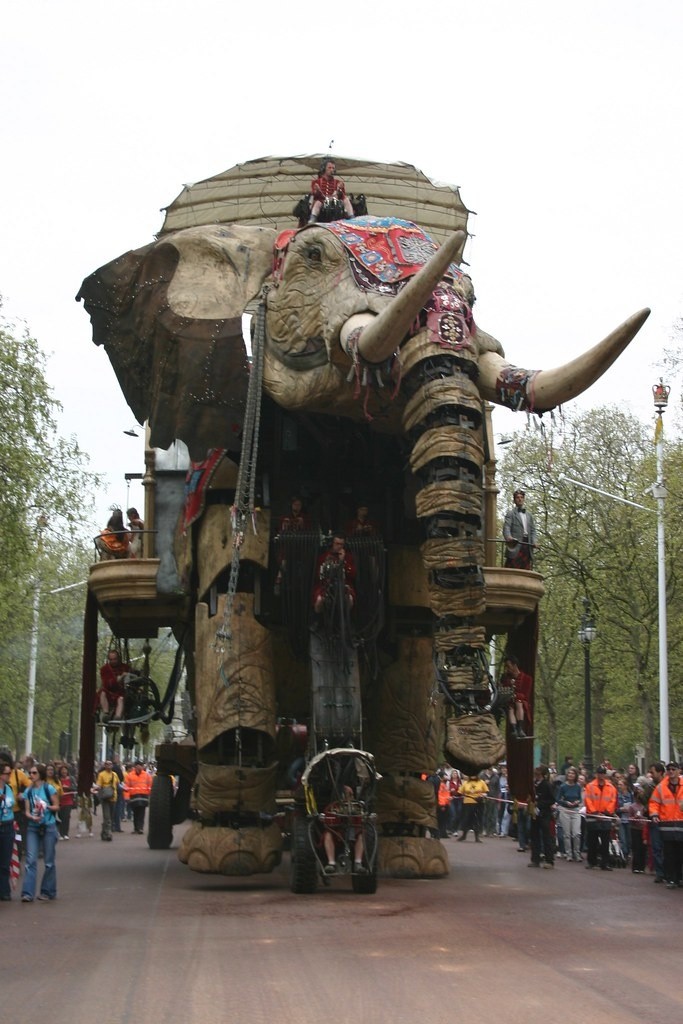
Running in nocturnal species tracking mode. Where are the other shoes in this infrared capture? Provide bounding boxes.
[107,837,112,841]
[353,863,366,873]
[37,894,49,900]
[324,863,336,873]
[64,835,69,840]
[434,829,683,890]
[131,831,138,834]
[102,714,119,732]
[2,895,12,902]
[58,835,63,841]
[88,832,93,836]
[21,895,32,902]
[138,830,143,835]
[309,621,319,634]
[76,833,81,837]
[121,735,134,750]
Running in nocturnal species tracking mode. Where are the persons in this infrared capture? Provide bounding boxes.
[308,534,356,633]
[344,499,378,538]
[21,764,60,902]
[502,656,532,737]
[0,747,159,861]
[93,650,140,722]
[429,756,683,890]
[324,785,368,873]
[99,508,144,562]
[273,498,312,595]
[503,490,540,571]
[0,762,14,901]
[308,158,355,224]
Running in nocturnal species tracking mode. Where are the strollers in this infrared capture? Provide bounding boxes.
[598,821,626,866]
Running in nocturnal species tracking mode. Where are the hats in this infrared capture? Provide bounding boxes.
[665,762,679,768]
[596,766,605,773]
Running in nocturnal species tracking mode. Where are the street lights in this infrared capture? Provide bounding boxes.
[578,598,596,770]
[560,379,670,768]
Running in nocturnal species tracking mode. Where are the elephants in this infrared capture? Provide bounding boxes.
[75,214,653,878]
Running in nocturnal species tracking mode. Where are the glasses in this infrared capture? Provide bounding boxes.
[3,772,11,775]
[598,772,605,774]
[29,771,39,775]
[666,768,676,771]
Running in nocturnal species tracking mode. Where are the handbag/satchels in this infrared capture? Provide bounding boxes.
[100,785,114,800]
[17,785,26,810]
